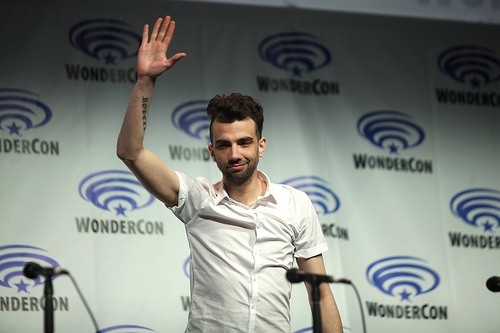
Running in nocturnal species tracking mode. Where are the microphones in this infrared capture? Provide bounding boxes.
[486,276,500,292]
[286,268,351,285]
[24,262,68,278]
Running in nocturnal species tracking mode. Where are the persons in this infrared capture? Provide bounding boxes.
[117,13,344,332]
